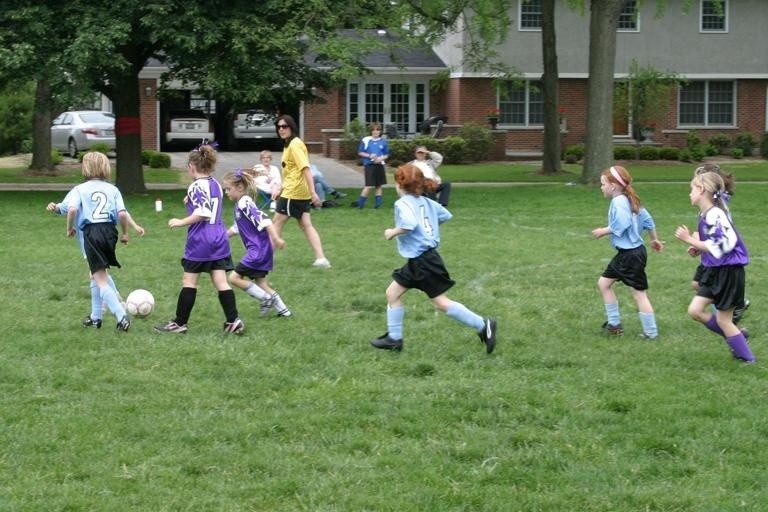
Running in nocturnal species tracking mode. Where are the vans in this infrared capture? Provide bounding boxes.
[227,101,285,146]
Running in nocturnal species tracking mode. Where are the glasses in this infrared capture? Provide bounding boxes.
[276,124,290,131]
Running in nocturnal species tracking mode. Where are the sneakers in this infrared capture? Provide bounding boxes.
[222,317,248,334]
[477,316,498,356]
[114,314,133,333]
[258,295,279,319]
[78,314,103,330]
[369,332,404,352]
[311,257,333,269]
[333,190,349,199]
[152,319,189,335]
[272,312,295,319]
[601,320,624,337]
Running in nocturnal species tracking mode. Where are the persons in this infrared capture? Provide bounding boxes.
[370,164,497,354]
[47,190,145,315]
[66,151,132,332]
[674,171,755,362]
[358,122,391,208]
[686,163,751,325]
[251,114,347,267]
[222,168,291,318]
[407,144,451,206]
[153,138,244,335]
[591,165,663,339]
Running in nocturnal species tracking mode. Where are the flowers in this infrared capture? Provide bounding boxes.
[557,106,564,123]
[639,121,656,132]
[483,108,501,120]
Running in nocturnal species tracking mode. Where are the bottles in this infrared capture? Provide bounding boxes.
[155,196,162,213]
[270,198,276,212]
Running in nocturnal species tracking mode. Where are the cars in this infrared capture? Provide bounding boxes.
[164,110,217,147]
[49,111,116,160]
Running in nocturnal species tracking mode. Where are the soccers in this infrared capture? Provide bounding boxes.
[126,289,155,318]
